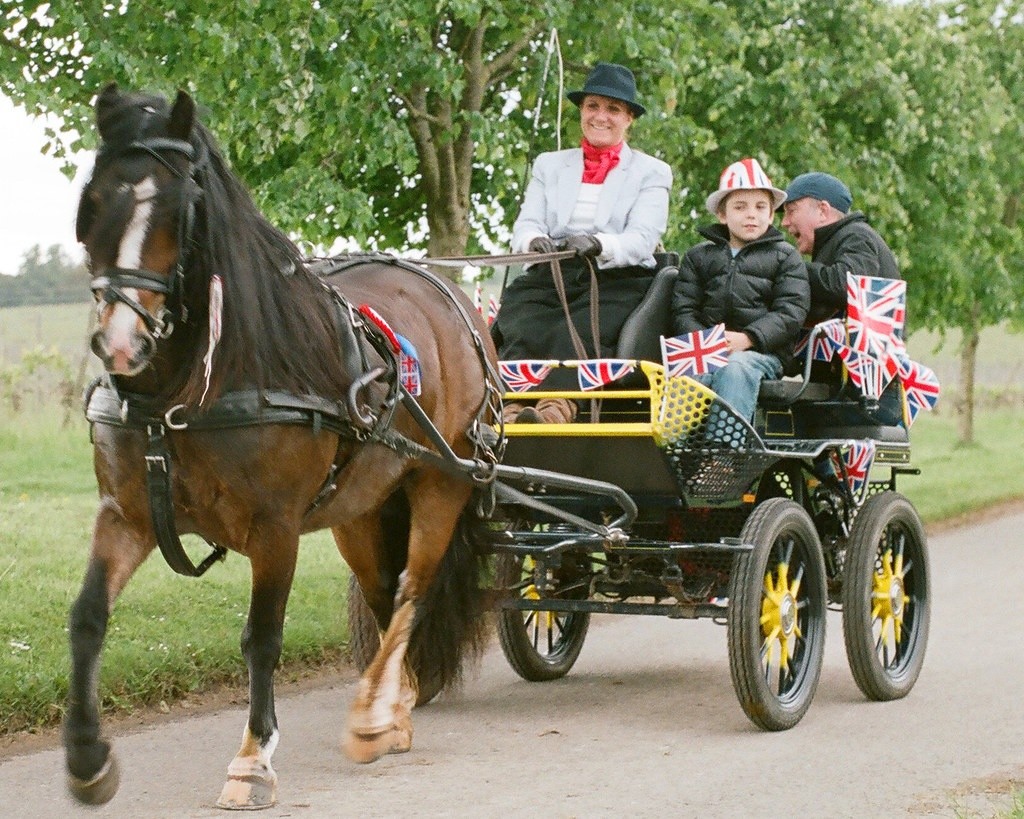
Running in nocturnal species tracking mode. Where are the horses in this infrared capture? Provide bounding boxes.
[64,81,502,810]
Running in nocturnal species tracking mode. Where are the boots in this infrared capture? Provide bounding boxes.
[515,397,577,424]
[498,403,526,425]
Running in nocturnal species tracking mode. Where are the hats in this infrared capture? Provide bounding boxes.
[568,63,646,119]
[705,158,788,215]
[774,171,853,213]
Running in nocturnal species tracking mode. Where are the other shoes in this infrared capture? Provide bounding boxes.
[688,459,734,494]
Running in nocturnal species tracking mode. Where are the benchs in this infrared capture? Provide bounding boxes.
[616,268,830,402]
[818,424,911,465]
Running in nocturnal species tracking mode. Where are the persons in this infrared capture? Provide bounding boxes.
[668,159,809,493]
[774,172,907,425]
[496,64,672,423]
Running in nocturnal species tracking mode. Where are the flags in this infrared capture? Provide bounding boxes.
[500,364,551,392]
[794,272,940,429]
[833,440,875,495]
[488,300,498,326]
[666,324,729,378]
[578,363,633,391]
[478,289,483,315]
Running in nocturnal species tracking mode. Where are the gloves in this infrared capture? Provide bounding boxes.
[564,235,602,256]
[529,235,557,254]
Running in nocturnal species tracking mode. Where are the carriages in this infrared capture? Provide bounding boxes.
[62,78,933,812]
